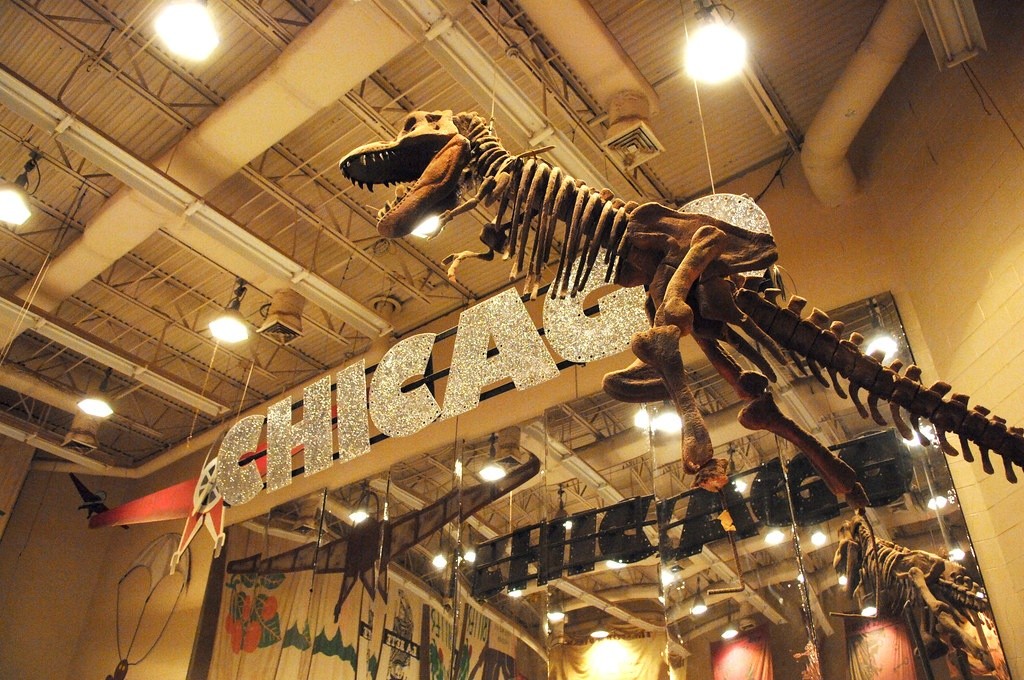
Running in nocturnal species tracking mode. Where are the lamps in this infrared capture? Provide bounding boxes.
[720,600,738,638]
[912,0,988,72]
[209,277,249,343]
[0,152,44,226]
[477,434,507,480]
[547,589,565,621]
[77,366,114,417]
[590,613,609,638]
[691,575,707,614]
